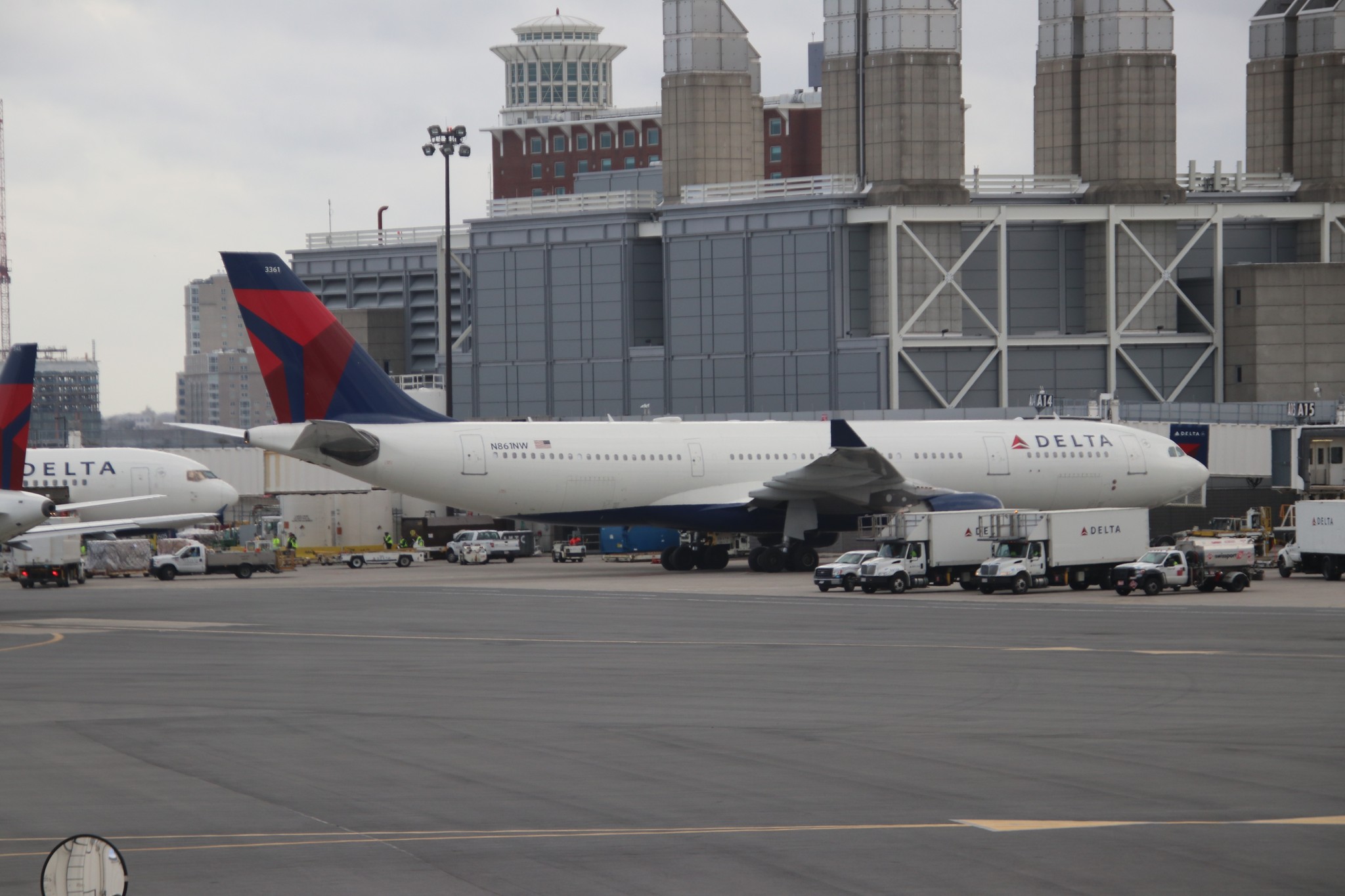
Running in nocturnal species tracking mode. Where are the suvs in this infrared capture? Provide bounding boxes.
[814,551,883,592]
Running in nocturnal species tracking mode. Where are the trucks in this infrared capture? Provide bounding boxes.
[973,509,1152,594]
[1273,499,1345,580]
[149,543,279,582]
[552,542,586,564]
[853,508,1042,596]
[16,533,86,590]
[1110,536,1256,596]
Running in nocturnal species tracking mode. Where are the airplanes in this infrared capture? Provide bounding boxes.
[161,257,1211,573]
[1,342,242,551]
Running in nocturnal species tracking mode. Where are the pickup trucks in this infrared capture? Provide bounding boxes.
[447,529,521,565]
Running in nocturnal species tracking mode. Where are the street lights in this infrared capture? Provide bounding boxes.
[421,124,474,418]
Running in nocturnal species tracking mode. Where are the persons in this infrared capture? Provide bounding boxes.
[284,532,297,558]
[253,533,260,541]
[904,545,917,559]
[570,534,581,545]
[271,533,280,547]
[1003,543,1020,557]
[410,533,424,548]
[396,534,409,549]
[1164,557,1178,566]
[184,547,197,558]
[383,532,393,549]
[1029,544,1040,559]
